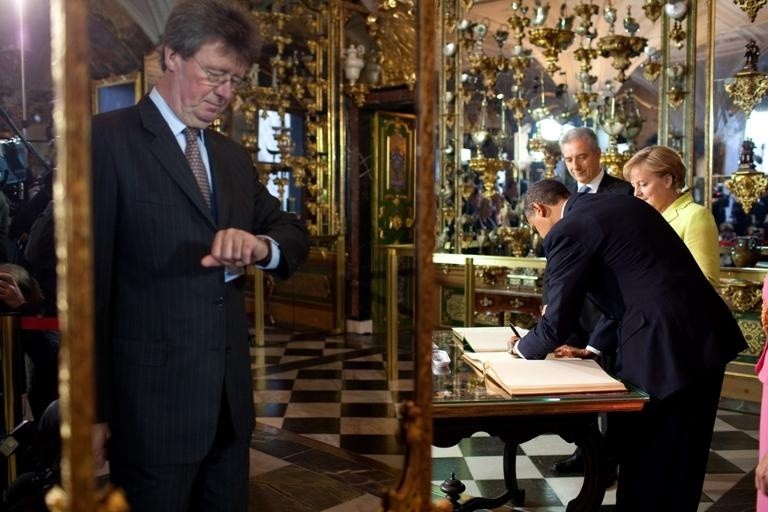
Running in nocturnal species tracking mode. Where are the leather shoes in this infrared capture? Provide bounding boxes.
[551,450,586,475]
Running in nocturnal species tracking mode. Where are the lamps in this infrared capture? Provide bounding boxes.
[338,42,383,87]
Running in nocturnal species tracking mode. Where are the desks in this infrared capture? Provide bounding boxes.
[421,315,650,511]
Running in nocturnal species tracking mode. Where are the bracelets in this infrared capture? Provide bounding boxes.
[509,339,520,358]
[252,237,272,267]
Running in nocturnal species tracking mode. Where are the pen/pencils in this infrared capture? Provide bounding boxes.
[509,323,521,339]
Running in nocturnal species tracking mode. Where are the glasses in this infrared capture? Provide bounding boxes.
[193,55,249,93]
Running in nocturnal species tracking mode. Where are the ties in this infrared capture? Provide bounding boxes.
[181,125,212,219]
[578,185,588,193]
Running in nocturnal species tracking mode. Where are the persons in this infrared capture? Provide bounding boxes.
[87,2,311,511]
[622,144,721,291]
[752,273,768,512]
[505,177,750,512]
[1,128,60,510]
[553,127,634,489]
